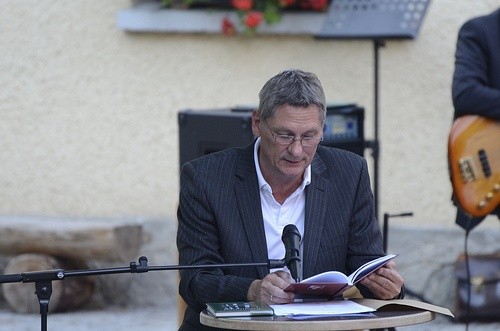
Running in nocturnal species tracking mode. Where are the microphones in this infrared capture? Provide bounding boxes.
[281,224,301,283]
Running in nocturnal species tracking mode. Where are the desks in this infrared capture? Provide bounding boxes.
[200,309,436,331]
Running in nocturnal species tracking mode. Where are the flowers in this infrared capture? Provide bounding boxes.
[221,1,332,38]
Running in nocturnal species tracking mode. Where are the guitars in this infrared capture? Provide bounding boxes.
[448,115,500,217]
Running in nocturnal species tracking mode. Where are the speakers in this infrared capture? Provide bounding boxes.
[177,106,365,171]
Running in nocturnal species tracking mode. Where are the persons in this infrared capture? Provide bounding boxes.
[451,6,500,232]
[176,69,405,331]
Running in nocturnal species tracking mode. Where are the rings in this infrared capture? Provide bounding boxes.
[270,294,274,302]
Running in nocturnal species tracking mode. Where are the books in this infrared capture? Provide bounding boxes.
[205,301,274,319]
[284,255,399,300]
[269,299,455,317]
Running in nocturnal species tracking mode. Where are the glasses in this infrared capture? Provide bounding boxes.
[262,115,323,146]
